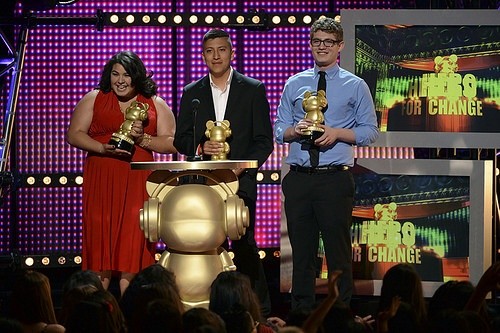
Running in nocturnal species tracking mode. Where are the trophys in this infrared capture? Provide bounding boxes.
[205,120,232,160]
[300,90,328,144]
[107,101,149,156]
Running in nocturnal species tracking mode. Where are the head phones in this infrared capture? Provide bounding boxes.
[137,171,250,243]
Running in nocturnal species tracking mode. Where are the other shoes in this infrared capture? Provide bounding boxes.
[285,309,313,328]
[325,303,350,325]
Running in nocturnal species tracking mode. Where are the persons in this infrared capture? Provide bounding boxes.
[66,51,176,296]
[0,250,275,333]
[267,263,500,333]
[173,29,275,323]
[274,17,379,316]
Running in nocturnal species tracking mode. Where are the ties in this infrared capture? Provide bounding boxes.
[308,70,327,168]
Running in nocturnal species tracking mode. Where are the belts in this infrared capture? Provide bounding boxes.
[290,164,349,174]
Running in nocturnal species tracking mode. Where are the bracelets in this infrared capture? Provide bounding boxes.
[139,133,151,148]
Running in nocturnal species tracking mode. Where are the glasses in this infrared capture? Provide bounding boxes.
[311,37,340,47]
